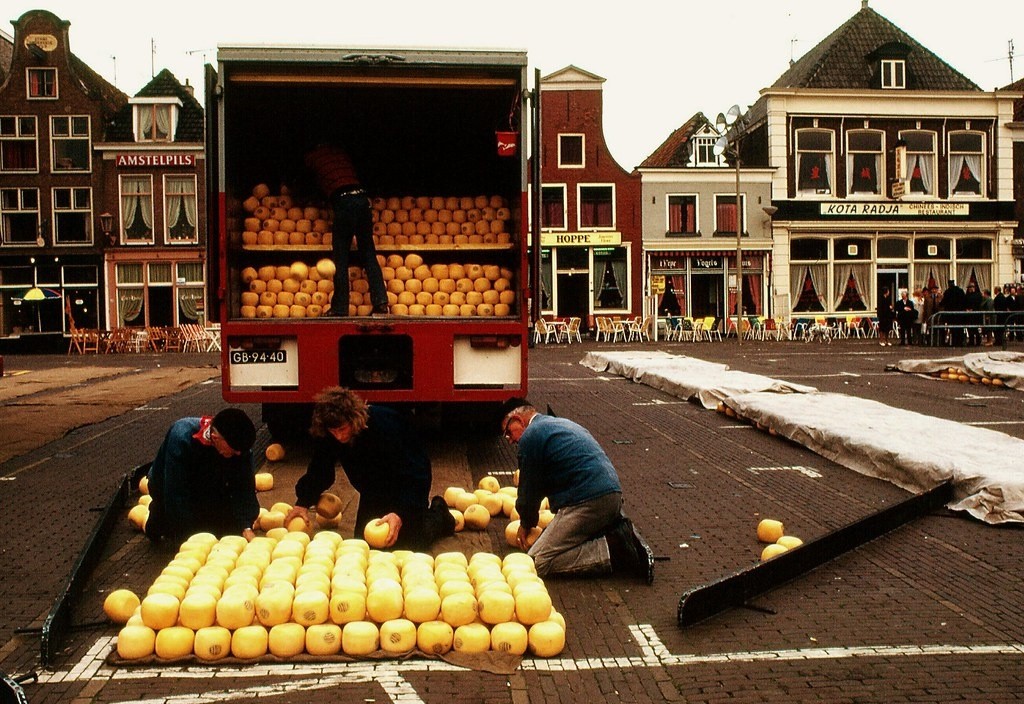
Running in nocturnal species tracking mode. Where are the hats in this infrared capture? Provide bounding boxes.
[215,408,256,451]
[500,397,533,418]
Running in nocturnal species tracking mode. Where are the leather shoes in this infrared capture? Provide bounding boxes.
[433,496,456,537]
[611,516,648,580]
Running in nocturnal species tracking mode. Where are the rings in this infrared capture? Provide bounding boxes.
[517,537,520,541]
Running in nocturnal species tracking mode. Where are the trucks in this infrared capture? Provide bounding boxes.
[202,42,546,450]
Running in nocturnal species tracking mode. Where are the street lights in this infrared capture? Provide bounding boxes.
[713,104,751,346]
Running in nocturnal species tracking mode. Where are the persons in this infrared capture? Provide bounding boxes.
[143,405,264,555]
[877,283,1024,353]
[494,393,654,584]
[743,306,747,315]
[664,308,671,319]
[301,133,394,316]
[282,386,455,552]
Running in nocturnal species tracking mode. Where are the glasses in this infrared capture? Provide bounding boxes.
[502,417,514,439]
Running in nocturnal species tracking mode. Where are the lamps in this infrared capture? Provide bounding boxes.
[100,212,113,236]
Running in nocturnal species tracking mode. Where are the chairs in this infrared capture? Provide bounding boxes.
[532,317,927,346]
[65,318,222,353]
